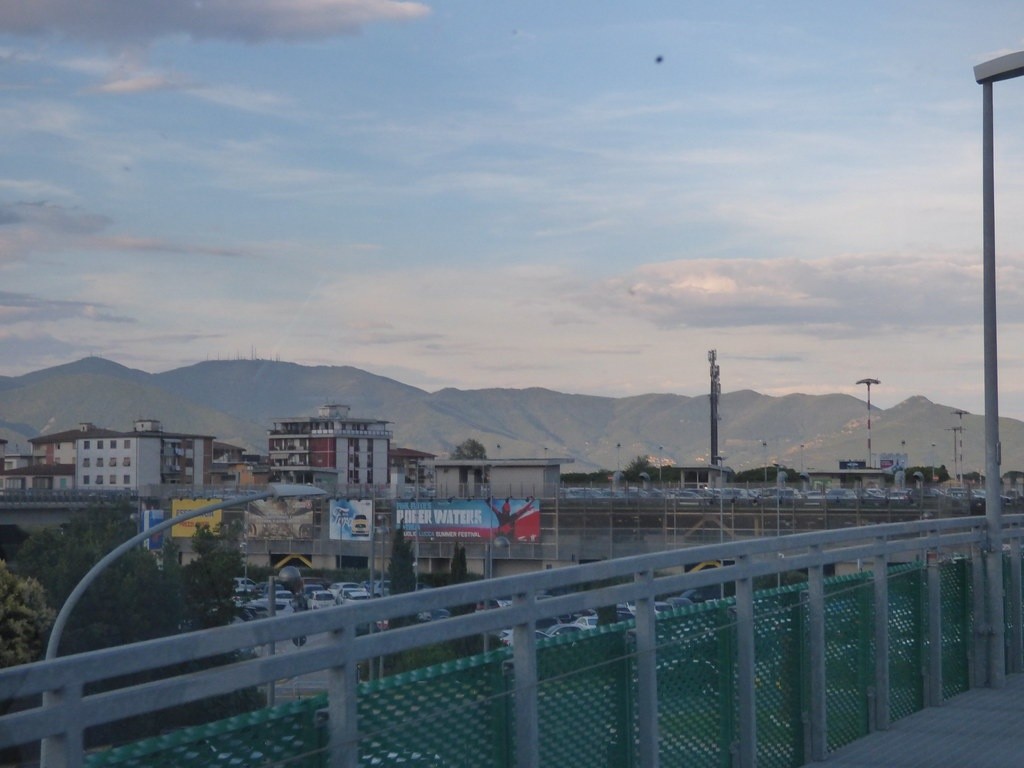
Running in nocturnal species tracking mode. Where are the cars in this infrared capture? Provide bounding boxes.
[558,486,1024,510]
[473,588,708,647]
[229,578,452,636]
[400,486,436,500]
[352,514,368,536]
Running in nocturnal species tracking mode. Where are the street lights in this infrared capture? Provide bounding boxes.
[762,440,768,484]
[856,376,882,467]
[951,409,970,485]
[930,442,936,483]
[659,445,663,486]
[616,442,621,487]
[947,427,965,485]
[800,444,805,473]
[40,482,328,767]
[973,52,1024,690]
